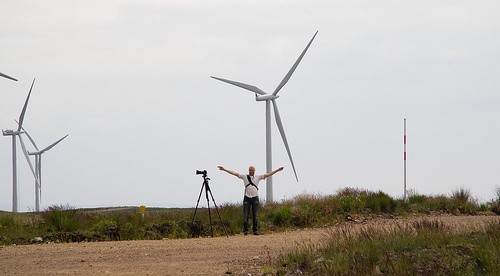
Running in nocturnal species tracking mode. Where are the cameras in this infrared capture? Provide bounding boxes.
[196,169,207,177]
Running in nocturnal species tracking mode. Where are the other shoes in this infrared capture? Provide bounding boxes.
[244,231,248,235]
[254,231,260,235]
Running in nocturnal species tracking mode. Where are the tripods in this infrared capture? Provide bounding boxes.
[186,178,229,238]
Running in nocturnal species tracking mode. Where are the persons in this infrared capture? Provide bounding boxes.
[216,165,285,236]
